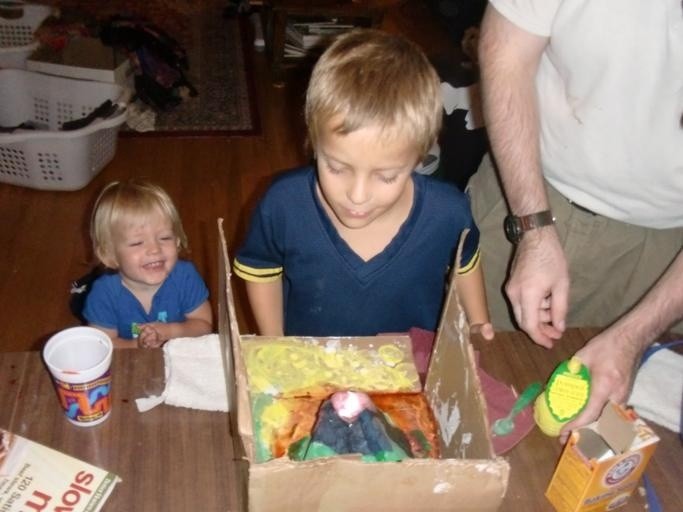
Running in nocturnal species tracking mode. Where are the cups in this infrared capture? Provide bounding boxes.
[43,326,114,427]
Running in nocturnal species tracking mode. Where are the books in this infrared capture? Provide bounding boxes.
[0,427,119,512]
[279,8,358,59]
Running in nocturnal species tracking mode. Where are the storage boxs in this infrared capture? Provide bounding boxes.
[217,217,511,512]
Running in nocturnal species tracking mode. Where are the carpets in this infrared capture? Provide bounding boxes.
[53,1,263,138]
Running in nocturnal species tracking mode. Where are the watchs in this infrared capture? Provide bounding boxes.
[503,209,554,244]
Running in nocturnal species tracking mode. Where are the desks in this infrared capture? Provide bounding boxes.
[1,327,683,512]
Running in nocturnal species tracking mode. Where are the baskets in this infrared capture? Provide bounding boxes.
[0,68,128,192]
[0,4,50,68]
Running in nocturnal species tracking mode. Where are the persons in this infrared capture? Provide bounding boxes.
[462,0,683,446]
[82,176,213,349]
[232,25,495,340]
